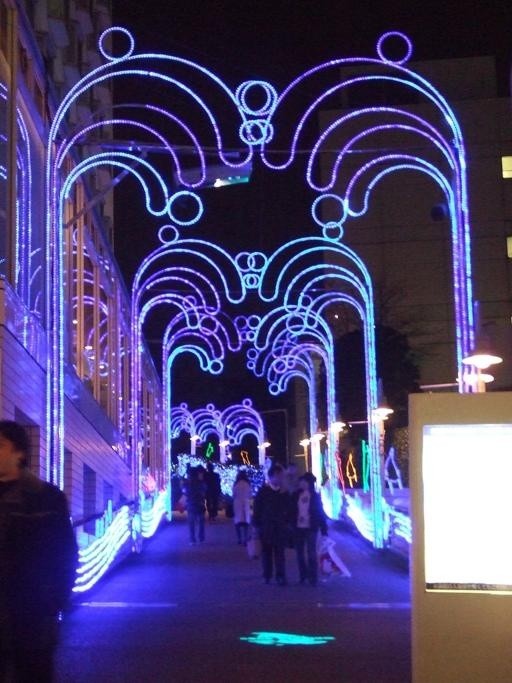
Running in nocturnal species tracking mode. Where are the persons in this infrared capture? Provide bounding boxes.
[171,462,329,585]
[1,419,78,681]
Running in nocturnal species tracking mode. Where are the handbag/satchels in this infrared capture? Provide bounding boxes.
[258,515,296,548]
[250,514,268,528]
[320,546,350,577]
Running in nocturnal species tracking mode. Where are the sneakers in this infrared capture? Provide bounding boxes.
[261,576,286,586]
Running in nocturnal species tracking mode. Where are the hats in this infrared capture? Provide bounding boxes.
[303,472,316,484]
